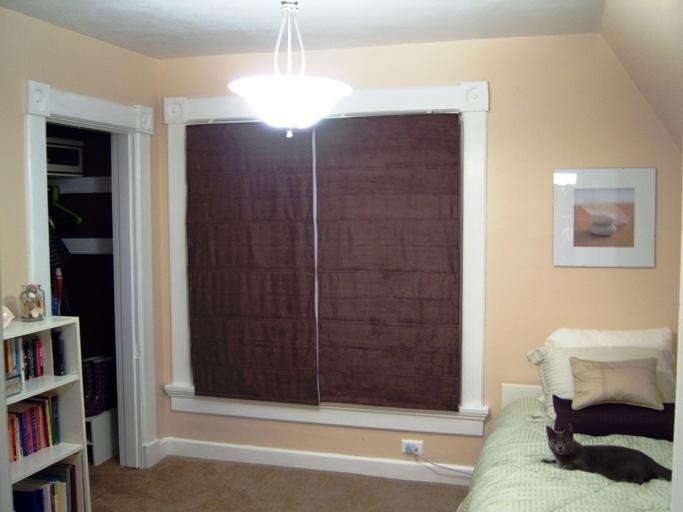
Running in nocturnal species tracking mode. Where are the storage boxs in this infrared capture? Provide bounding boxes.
[45,136,83,177]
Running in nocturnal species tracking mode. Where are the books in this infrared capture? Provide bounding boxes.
[3,329,78,512]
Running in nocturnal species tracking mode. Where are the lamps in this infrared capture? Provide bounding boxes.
[225,0,355,139]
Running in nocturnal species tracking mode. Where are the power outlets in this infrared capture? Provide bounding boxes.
[401,439,423,455]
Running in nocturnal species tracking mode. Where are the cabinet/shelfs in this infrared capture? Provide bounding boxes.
[0,313,91,511]
[85,406,117,467]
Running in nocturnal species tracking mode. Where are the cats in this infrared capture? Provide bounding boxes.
[541,424,672,485]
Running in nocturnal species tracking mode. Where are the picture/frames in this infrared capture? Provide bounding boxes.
[552,168,655,268]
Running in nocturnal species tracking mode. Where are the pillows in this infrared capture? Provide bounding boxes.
[526,327,677,442]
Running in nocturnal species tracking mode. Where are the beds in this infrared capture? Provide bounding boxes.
[456,381,676,511]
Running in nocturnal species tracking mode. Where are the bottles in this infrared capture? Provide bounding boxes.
[20,283,46,323]
